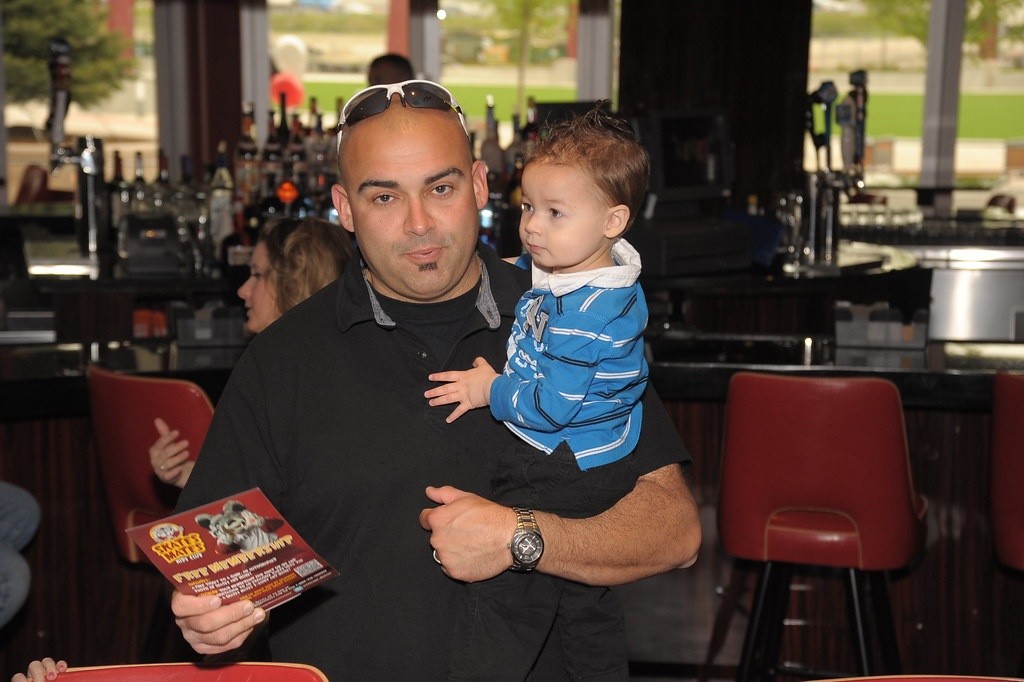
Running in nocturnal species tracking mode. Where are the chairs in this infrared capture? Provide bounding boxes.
[716,372,931,682]
[79,364,215,664]
[993,370,1024,578]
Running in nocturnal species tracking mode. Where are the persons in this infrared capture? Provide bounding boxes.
[367,54,414,87]
[425,104,650,682]
[171,78,700,682]
[148,218,364,488]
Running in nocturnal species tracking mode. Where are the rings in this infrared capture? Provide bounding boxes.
[160,464,166,471]
[432,550,441,564]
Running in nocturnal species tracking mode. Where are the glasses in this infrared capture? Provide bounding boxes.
[333,80,471,155]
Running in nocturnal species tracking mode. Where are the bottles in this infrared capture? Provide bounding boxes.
[106,90,759,288]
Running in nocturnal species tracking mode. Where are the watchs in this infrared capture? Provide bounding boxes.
[509,506,544,573]
